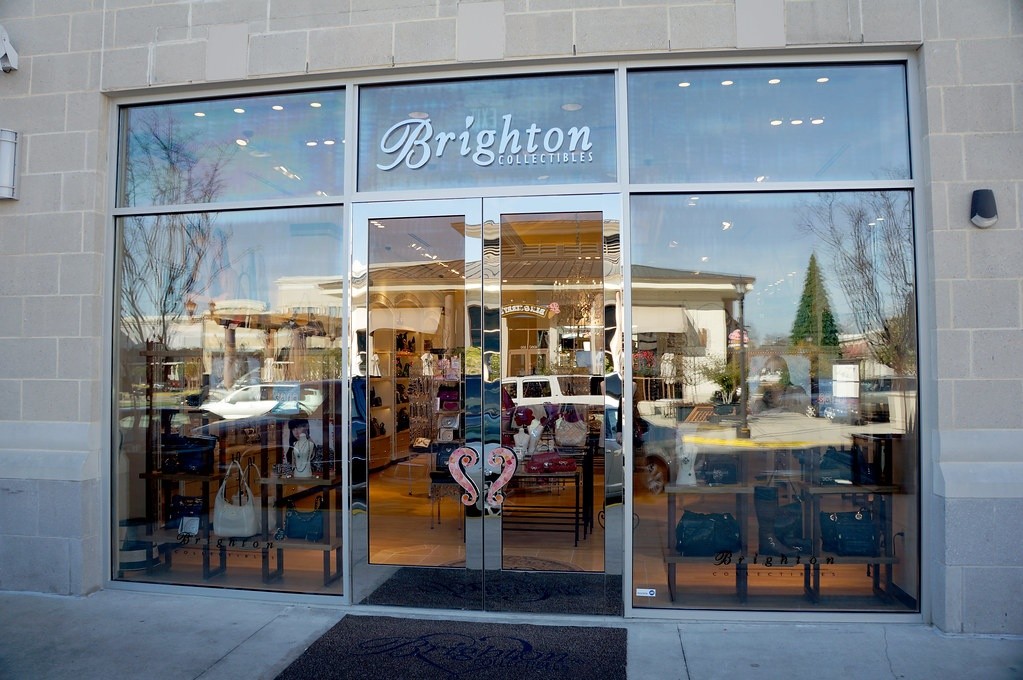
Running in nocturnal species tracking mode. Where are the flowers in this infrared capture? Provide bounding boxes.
[633,352,660,378]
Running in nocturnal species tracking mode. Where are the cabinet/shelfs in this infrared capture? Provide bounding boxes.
[663,261,899,603]
[118,319,426,587]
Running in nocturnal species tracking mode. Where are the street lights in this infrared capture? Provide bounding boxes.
[732,275,750,438]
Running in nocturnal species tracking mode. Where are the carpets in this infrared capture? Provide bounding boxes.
[359,566,625,618]
[273,611,628,680]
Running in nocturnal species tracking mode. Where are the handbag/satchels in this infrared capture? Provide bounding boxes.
[284,497,323,542]
[432,386,459,470]
[161,433,216,475]
[232,463,276,534]
[213,461,256,537]
[554,400,587,447]
[703,461,736,485]
[672,512,737,555]
[525,426,577,473]
[820,507,881,555]
[164,496,204,530]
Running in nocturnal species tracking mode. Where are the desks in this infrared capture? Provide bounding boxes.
[429,469,580,550]
[523,447,595,540]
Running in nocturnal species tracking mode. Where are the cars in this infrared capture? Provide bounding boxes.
[500,373,619,422]
[153,384,164,388]
[439,404,683,500]
[780,376,917,424]
[231,365,283,390]
[200,384,324,419]
[119,406,215,452]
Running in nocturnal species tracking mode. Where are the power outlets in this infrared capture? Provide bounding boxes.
[900,527,908,547]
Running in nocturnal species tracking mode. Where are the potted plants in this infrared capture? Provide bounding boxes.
[688,350,752,415]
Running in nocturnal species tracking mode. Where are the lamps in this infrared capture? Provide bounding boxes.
[969,188,998,229]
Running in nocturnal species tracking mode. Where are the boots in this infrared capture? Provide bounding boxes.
[754,486,801,558]
